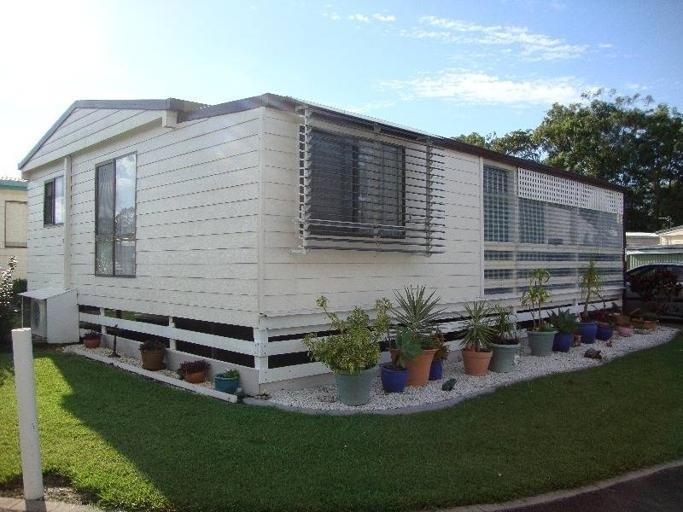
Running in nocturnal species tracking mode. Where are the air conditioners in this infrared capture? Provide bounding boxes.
[30,297,47,338]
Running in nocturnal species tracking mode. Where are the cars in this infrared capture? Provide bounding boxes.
[624,262,683,322]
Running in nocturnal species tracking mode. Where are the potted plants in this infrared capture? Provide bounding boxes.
[176,359,211,384]
[385,284,450,389]
[82,325,102,347]
[594,299,659,342]
[422,324,449,380]
[301,295,392,407]
[546,308,582,352]
[214,369,240,393]
[451,298,500,378]
[521,270,558,357]
[574,258,608,344]
[138,339,166,371]
[379,329,422,393]
[486,303,521,374]
